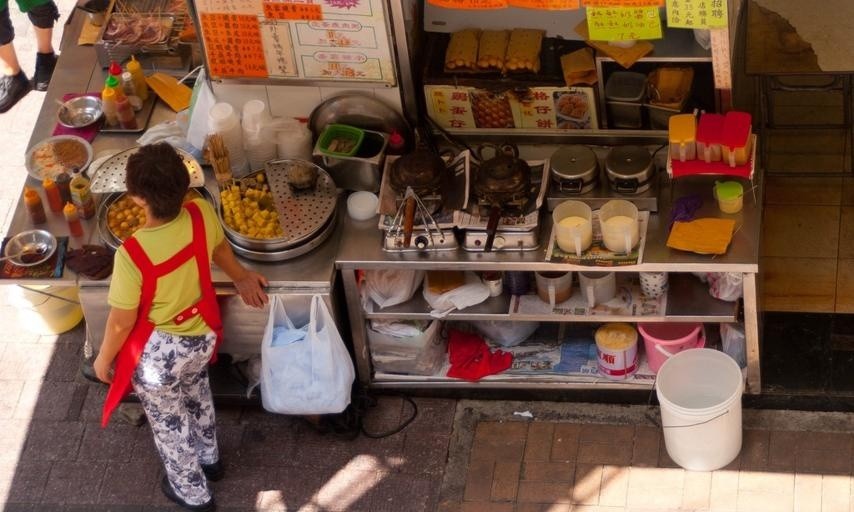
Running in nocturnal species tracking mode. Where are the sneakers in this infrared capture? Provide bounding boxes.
[161,474,216,512]
[201,458,225,481]
[0,75,31,112]
[34,53,57,91]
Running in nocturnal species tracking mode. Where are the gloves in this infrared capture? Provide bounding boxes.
[447,332,514,381]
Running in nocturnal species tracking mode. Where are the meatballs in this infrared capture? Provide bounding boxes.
[470,94,515,128]
[108,194,147,241]
[221,174,286,239]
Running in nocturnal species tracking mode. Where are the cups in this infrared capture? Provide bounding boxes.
[711,180,744,214]
[596,201,643,259]
[535,271,574,309]
[578,271,617,309]
[553,200,595,263]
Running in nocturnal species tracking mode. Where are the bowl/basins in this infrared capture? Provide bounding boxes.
[277,120,314,160]
[4,229,57,268]
[242,98,276,171]
[54,95,104,129]
[207,101,250,179]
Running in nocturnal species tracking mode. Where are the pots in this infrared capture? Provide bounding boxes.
[602,145,658,197]
[476,143,531,252]
[393,149,439,249]
[105,189,203,247]
[219,161,341,262]
[549,145,601,196]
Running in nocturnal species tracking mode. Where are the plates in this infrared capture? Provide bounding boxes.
[22,135,94,182]
[555,92,591,123]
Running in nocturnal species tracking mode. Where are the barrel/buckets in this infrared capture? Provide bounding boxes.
[18,284,84,335]
[643,349,744,473]
[636,321,706,373]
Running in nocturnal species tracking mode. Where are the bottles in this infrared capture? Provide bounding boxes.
[21,166,96,239]
[102,52,150,129]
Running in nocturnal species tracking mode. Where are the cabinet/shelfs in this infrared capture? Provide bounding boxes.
[337,140,763,394]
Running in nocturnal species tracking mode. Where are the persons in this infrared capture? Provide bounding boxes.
[1,0,64,111]
[95,142,269,508]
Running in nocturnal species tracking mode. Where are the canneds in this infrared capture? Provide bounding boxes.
[639,272,668,298]
[483,275,503,297]
[594,322,640,379]
